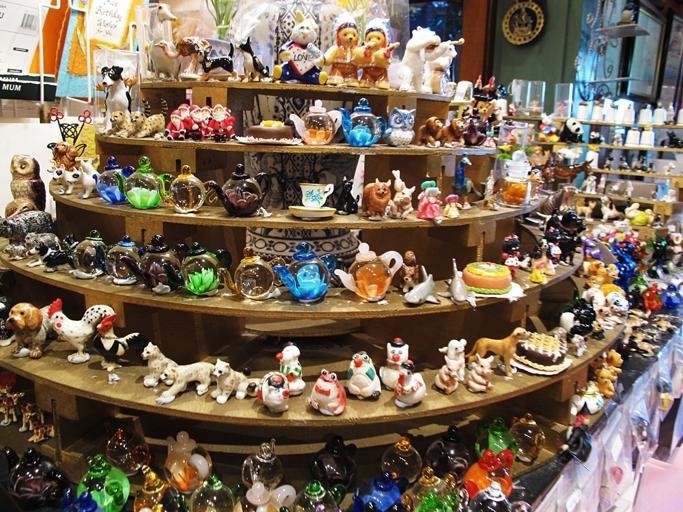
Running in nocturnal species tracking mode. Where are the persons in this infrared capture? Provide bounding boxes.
[164,103,236,143]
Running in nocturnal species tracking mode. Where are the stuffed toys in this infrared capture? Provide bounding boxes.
[591,349,623,373]
[593,368,615,398]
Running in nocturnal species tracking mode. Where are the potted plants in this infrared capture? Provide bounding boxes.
[203,0,239,60]
